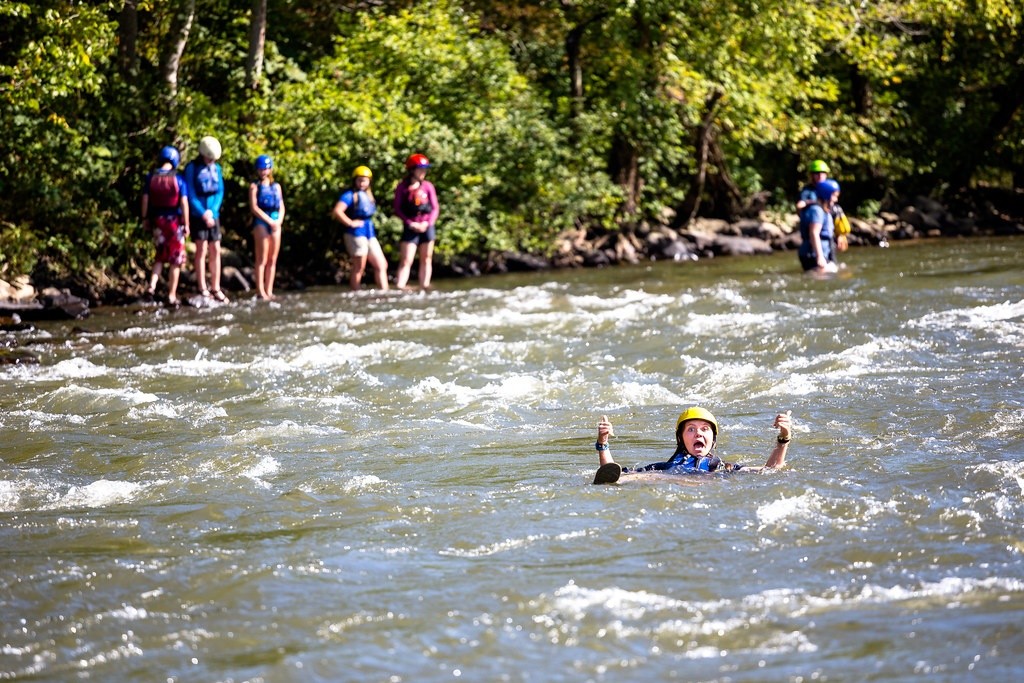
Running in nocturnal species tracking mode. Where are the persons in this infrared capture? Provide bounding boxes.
[249,154,285,303]
[140,145,190,308]
[184,136,229,307]
[797,160,852,276]
[393,154,440,291]
[334,166,388,291]
[593,405,793,485]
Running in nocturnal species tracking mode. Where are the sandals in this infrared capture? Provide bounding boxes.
[197,287,214,300]
[210,289,230,304]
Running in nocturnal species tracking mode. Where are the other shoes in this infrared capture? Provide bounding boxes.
[593,463,621,485]
[166,299,182,306]
[145,286,156,296]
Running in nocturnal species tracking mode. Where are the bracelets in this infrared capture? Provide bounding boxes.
[777,435,792,444]
[595,440,610,451]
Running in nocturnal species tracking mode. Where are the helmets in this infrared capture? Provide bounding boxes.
[255,155,273,169]
[405,154,430,169]
[351,166,373,178]
[816,179,841,201]
[676,407,719,439]
[809,159,830,173]
[158,146,179,169]
[200,136,221,160]
